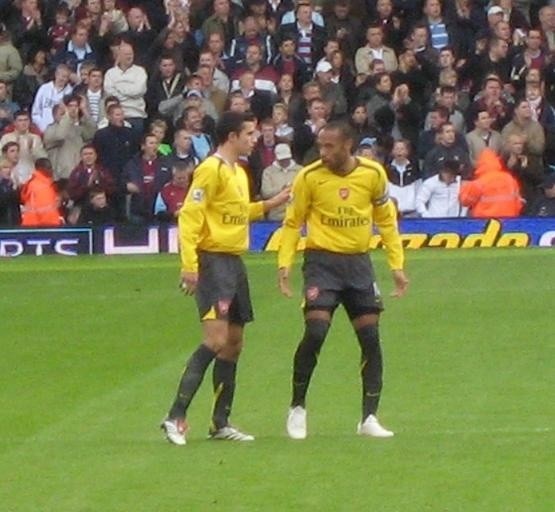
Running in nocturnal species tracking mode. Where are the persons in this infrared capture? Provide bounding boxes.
[364,72,397,133]
[231,43,280,96]
[515,67,555,125]
[429,86,442,108]
[47,8,76,61]
[11,0,51,64]
[325,0,354,50]
[374,135,394,165]
[46,104,66,132]
[71,61,97,97]
[0,110,48,185]
[375,79,428,139]
[357,59,385,107]
[430,46,469,93]
[464,108,501,167]
[470,36,514,95]
[500,97,546,168]
[76,0,101,29]
[509,26,555,87]
[82,69,111,123]
[208,111,258,203]
[465,5,507,67]
[147,120,172,157]
[394,21,439,70]
[192,48,229,96]
[12,42,51,105]
[67,145,117,207]
[243,0,275,35]
[204,30,232,77]
[389,49,431,105]
[328,50,358,109]
[278,3,329,79]
[160,111,293,447]
[154,161,194,225]
[277,120,409,440]
[171,88,211,127]
[312,37,341,78]
[233,70,281,126]
[157,74,219,126]
[173,21,201,74]
[0,78,22,131]
[55,23,99,74]
[473,71,516,105]
[121,3,158,67]
[354,25,398,89]
[294,98,329,164]
[273,37,308,93]
[280,0,325,28]
[467,78,513,133]
[196,65,227,119]
[447,0,476,57]
[528,175,555,218]
[101,0,129,37]
[312,60,347,123]
[19,158,63,228]
[274,72,302,118]
[31,63,74,132]
[260,143,305,222]
[295,79,322,123]
[77,189,126,226]
[270,101,295,148]
[493,20,513,46]
[538,6,555,54]
[156,129,201,193]
[416,0,462,51]
[417,158,469,219]
[423,86,468,135]
[71,12,112,63]
[225,96,249,114]
[96,96,133,130]
[496,0,530,34]
[347,103,381,154]
[358,144,376,162]
[360,0,394,47]
[44,94,99,193]
[303,124,326,166]
[103,44,149,136]
[416,102,469,169]
[386,13,411,48]
[120,133,172,226]
[92,105,144,178]
[0,22,22,97]
[385,139,423,218]
[0,156,21,228]
[421,123,474,181]
[181,106,216,164]
[249,119,297,203]
[2,104,44,142]
[232,10,282,68]
[143,53,188,119]
[158,0,199,32]
[502,134,543,198]
[148,8,186,74]
[459,148,527,219]
[201,0,241,56]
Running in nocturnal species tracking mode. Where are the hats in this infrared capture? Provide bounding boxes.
[487,5,504,14]
[316,62,333,73]
[274,144,292,160]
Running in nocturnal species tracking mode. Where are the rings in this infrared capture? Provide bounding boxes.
[182,282,186,289]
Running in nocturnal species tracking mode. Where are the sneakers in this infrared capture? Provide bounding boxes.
[286,406,307,439]
[161,416,188,446]
[355,415,395,437]
[206,426,254,441]
